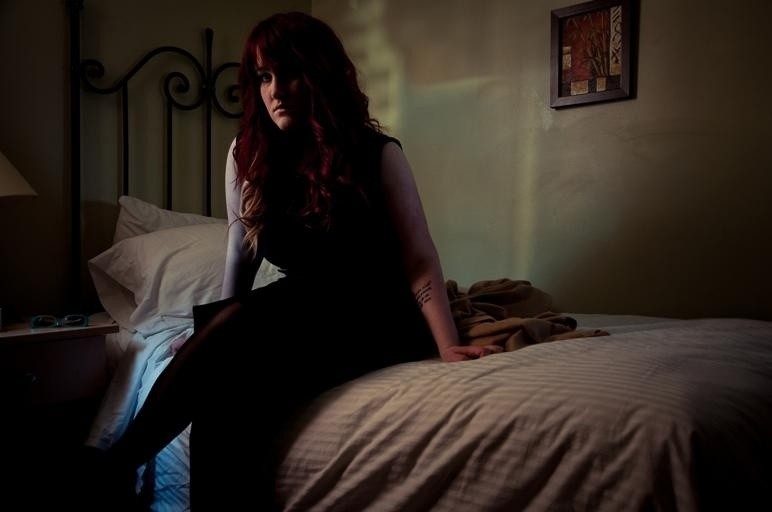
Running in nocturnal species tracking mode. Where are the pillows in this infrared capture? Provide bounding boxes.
[87,190,280,335]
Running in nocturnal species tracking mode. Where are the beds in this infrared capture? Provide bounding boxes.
[70,1,769,512]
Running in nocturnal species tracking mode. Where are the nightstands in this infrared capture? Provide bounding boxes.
[1,313,122,412]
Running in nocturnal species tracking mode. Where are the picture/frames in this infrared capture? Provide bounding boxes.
[549,1,641,108]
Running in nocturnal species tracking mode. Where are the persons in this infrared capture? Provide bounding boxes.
[43,9,505,511]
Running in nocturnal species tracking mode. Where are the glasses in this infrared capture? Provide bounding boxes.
[30,314,91,329]
